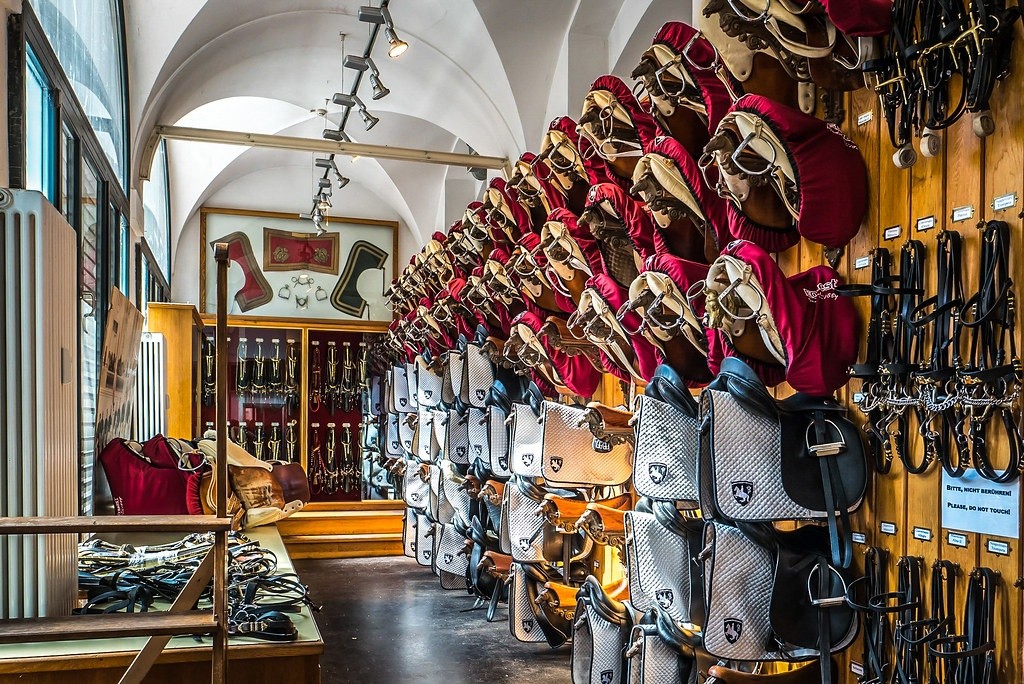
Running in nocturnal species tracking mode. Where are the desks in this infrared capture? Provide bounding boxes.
[0,524,324,684]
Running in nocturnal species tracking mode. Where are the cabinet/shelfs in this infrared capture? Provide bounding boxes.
[146,302,409,510]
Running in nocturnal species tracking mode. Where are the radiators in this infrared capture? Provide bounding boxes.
[134,334,167,442]
[0,188,79,621]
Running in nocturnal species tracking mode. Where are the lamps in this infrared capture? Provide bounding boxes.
[312,177,332,210]
[344,55,390,100]
[333,93,379,132]
[355,5,408,58]
[322,129,359,163]
[316,159,350,189]
[298,212,327,239]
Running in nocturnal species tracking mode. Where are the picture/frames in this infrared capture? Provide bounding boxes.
[199,208,399,324]
